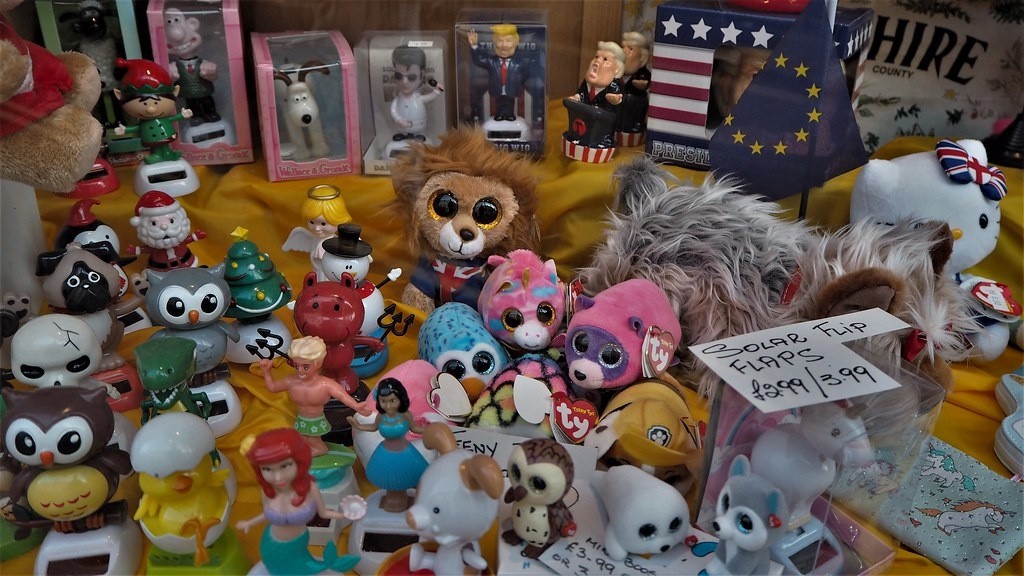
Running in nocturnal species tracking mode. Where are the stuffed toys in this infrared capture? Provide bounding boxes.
[388,121,1011,483]
[0,0,103,194]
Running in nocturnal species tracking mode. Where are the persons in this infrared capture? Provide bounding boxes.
[346,378,429,513]
[569,41,625,148]
[301,185,351,282]
[126,190,207,275]
[390,44,445,142]
[469,24,530,123]
[111,58,194,163]
[617,32,651,133]
[241,428,367,576]
[260,336,370,458]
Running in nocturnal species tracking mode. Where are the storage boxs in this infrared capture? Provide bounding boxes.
[453,7,550,162]
[685,301,946,576]
[354,29,452,177]
[146,0,255,166]
[35,0,152,155]
[645,0,875,173]
[250,28,363,181]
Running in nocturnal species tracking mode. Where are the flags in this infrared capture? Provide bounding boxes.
[709,0,869,206]
